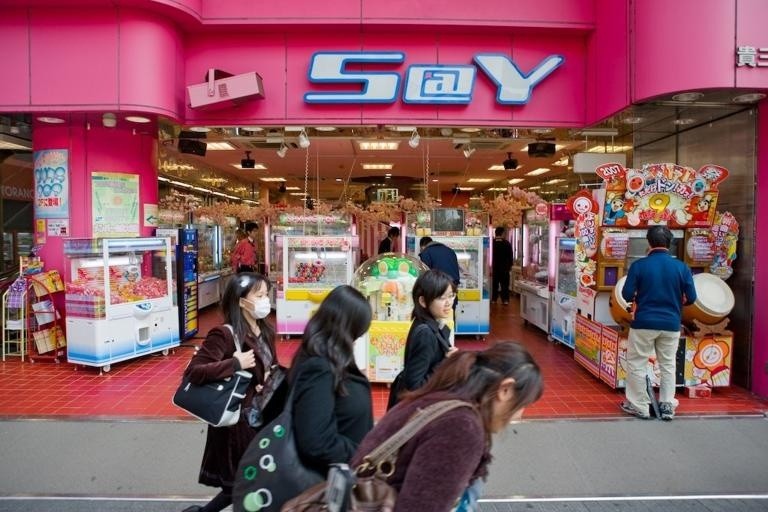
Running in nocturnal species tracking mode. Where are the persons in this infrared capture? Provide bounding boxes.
[621,225,695,420]
[375,225,400,256]
[417,236,460,333]
[335,337,545,511]
[283,285,377,479]
[230,221,259,275]
[400,269,481,511]
[179,269,288,512]
[490,228,512,305]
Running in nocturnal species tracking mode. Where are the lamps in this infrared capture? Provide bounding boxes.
[336,127,426,203]
[158,129,315,203]
[456,140,567,203]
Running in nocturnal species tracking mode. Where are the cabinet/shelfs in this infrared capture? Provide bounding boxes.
[264,218,359,343]
[357,210,493,343]
[157,209,248,341]
[486,210,581,352]
[64,237,182,375]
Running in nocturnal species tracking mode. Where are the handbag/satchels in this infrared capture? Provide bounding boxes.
[233,410,325,511]
[172,325,252,426]
[281,462,397,512]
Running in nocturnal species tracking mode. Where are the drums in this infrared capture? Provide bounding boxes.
[609,272,735,329]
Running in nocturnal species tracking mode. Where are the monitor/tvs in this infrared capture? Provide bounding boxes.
[624,238,679,274]
[435,209,464,231]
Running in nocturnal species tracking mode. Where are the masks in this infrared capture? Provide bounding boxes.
[239,297,271,319]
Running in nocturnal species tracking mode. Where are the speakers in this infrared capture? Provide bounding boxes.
[503,159,517,169]
[528,143,555,157]
[241,159,255,168]
[179,139,206,156]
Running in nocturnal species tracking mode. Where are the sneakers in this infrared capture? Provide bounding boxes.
[620,400,650,418]
[661,402,675,419]
